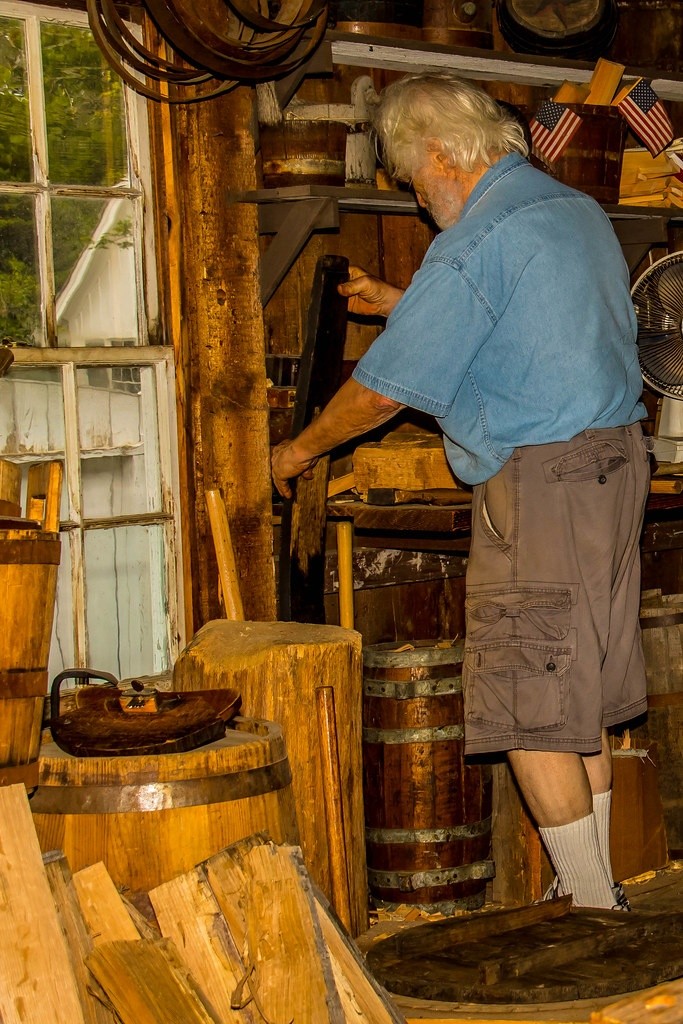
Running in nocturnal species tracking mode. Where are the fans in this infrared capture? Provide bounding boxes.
[630,250,683,464]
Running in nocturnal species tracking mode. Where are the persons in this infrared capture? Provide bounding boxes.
[270,69,652,914]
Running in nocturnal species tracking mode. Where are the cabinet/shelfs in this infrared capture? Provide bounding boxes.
[231,29,683,306]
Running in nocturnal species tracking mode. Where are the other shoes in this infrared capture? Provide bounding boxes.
[530,875,632,911]
